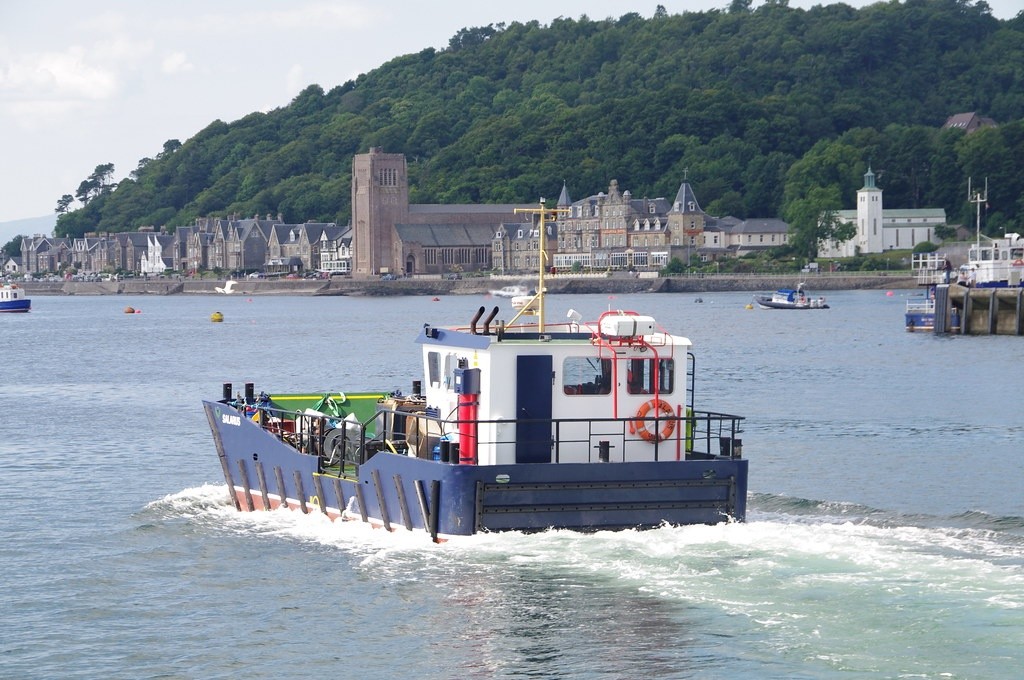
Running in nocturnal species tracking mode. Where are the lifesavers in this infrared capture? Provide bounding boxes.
[1013,258,1024,266]
[637,398,675,444]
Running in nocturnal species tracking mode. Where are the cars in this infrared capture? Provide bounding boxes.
[447,273,462,280]
[382,274,397,281]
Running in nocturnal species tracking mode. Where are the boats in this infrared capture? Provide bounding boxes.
[752,260,830,310]
[0,280,32,314]
[486,284,530,298]
[201,197,752,545]
[953,176,1023,289]
[215,279,242,294]
[905,250,954,332]
[511,292,540,310]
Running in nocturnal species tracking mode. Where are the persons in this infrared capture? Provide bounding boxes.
[941,257,953,285]
[957,272,967,286]
[968,267,977,288]
